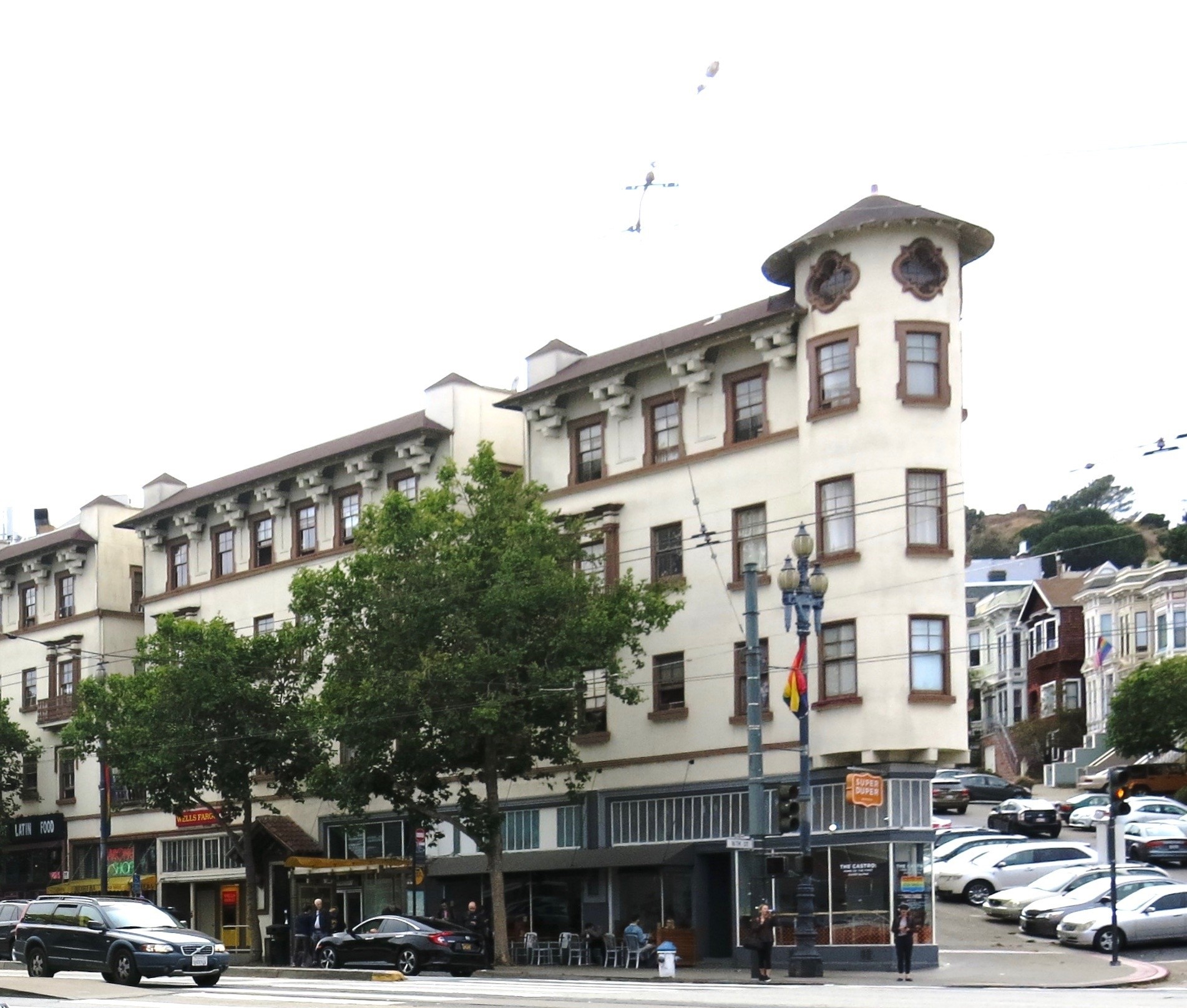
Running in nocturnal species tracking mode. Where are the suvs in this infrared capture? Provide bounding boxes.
[0,899,36,960]
[11,894,231,988]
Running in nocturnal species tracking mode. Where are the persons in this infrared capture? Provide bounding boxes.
[624,914,657,968]
[580,922,610,966]
[436,899,494,969]
[663,919,677,929]
[751,904,777,983]
[892,905,916,981]
[382,901,402,915]
[293,899,346,968]
[508,916,530,942]
[366,922,381,934]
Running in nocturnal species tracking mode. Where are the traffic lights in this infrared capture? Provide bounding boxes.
[1107,768,1133,816]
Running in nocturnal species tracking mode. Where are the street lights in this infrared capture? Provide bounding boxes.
[774,522,835,976]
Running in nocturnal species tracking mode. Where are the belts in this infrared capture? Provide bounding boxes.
[314,928,321,930]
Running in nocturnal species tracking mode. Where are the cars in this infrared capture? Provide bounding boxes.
[1056,792,1111,826]
[1068,794,1187,830]
[315,914,488,979]
[931,778,969,815]
[921,816,1185,938]
[1122,823,1187,869]
[1056,882,1187,954]
[934,768,1034,802]
[987,799,1063,840]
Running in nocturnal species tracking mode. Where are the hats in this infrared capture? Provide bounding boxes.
[901,904,909,910]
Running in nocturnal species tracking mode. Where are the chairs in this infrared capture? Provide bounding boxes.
[523,931,589,967]
[603,933,623,968]
[624,933,646,969]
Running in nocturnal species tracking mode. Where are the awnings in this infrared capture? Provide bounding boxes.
[46,875,156,895]
[427,842,695,876]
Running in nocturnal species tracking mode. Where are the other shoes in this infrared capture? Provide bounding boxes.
[481,964,495,970]
[759,975,771,982]
[897,978,903,981]
[301,962,306,967]
[292,962,296,967]
[905,978,912,981]
